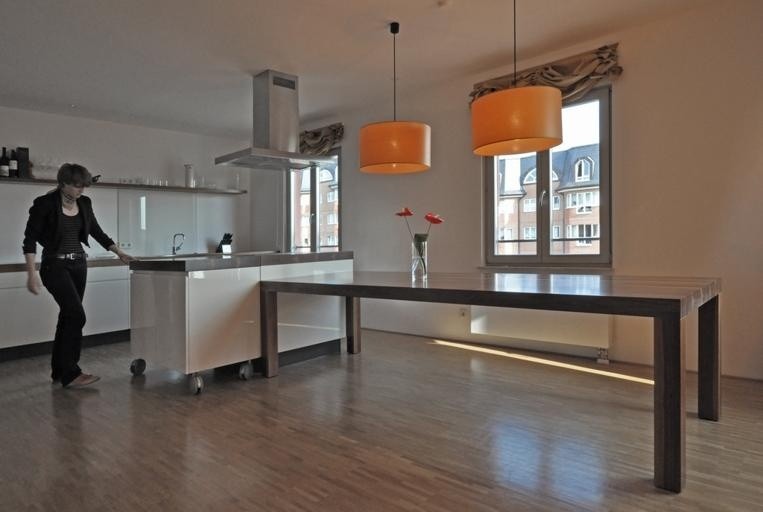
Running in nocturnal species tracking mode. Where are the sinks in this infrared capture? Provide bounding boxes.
[134,253,220,259]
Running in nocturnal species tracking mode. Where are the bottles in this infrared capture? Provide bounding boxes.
[0,146,18,180]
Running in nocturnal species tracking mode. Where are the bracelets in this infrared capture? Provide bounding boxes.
[110,245,119,252]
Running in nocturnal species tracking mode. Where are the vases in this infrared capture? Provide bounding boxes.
[410,241,428,274]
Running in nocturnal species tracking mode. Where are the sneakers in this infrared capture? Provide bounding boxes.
[64,374,101,387]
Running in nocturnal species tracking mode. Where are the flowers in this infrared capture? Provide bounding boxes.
[394,206,445,274]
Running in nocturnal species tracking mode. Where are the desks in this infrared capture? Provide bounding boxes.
[258,275,725,494]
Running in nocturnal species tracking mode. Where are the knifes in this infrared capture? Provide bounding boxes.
[221,231,234,245]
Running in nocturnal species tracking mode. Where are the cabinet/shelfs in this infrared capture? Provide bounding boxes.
[0,265,132,349]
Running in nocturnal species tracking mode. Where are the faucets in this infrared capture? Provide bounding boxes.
[171,233,186,254]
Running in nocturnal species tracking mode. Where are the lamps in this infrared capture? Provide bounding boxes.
[468,2,565,158]
[359,20,431,174]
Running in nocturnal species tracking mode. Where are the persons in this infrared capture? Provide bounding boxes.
[22,162,134,388]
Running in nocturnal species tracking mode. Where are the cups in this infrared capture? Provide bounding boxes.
[142,174,170,187]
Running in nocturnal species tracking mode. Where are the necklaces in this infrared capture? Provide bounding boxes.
[61,191,74,204]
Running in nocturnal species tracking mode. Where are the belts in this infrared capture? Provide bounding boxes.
[50,253,86,261]
